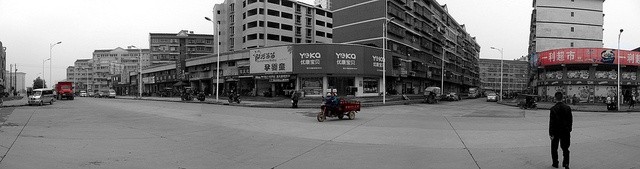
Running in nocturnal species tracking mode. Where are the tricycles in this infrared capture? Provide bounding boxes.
[517,93,537,110]
[317,95,360,122]
[423,87,440,103]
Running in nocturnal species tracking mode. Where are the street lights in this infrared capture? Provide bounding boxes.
[616,29,624,111]
[441,47,455,95]
[43,58,54,88]
[50,41,63,88]
[205,16,220,101]
[83,69,88,92]
[489,46,503,102]
[381,17,395,105]
[132,45,142,98]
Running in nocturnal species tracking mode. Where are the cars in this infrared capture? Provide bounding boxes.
[445,92,459,100]
[87,91,94,97]
[487,93,497,102]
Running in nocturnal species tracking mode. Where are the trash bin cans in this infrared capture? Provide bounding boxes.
[606,100,617,111]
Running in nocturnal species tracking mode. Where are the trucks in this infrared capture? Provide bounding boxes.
[55,80,74,100]
[468,87,480,98]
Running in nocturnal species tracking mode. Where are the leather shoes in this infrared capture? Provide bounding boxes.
[552,164,558,168]
[565,166,570,169]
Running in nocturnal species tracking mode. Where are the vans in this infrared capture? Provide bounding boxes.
[106,89,116,98]
[28,88,54,105]
[94,89,108,97]
[79,90,86,97]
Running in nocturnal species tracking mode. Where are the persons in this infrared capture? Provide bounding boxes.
[324,92,337,117]
[628,94,636,109]
[291,91,298,108]
[549,92,573,168]
[198,90,206,104]
[606,95,611,111]
[193,88,198,97]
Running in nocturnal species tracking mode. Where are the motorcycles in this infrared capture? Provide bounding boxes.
[228,91,241,104]
